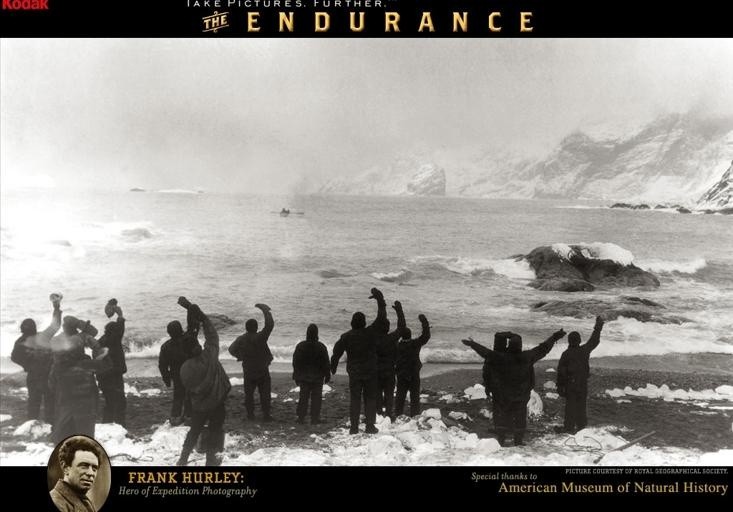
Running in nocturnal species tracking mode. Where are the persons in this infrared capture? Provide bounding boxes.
[459,324,567,447]
[330,286,431,434]
[228,304,276,421]
[49,435,101,512]
[552,313,617,434]
[158,295,203,429]
[174,303,233,466]
[9,290,128,448]
[291,323,332,424]
